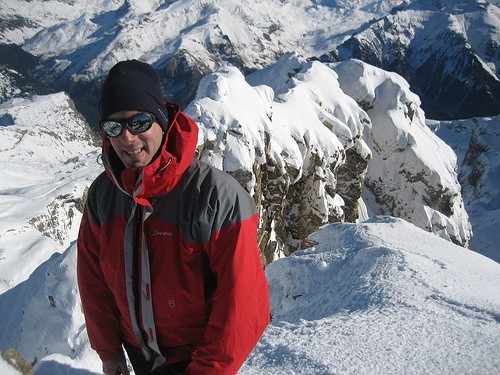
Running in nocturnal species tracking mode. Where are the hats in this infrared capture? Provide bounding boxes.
[99,59,168,130]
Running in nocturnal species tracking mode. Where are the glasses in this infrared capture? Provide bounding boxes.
[99,112,157,138]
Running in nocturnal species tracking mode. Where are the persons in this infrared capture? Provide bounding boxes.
[77,59,272,375]
